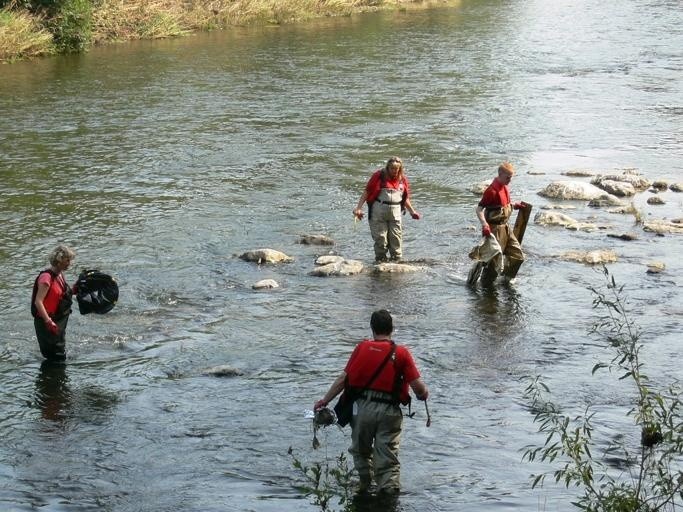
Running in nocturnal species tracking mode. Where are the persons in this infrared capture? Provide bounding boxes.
[29,245,79,364]
[474,161,524,291]
[312,308,429,499]
[351,155,420,265]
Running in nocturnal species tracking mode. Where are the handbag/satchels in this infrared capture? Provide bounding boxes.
[334,392,352,426]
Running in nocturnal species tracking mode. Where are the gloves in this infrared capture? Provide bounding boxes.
[46,318,58,333]
[483,225,491,236]
[313,399,326,411]
[417,390,428,401]
[514,202,526,209]
[412,213,419,220]
[353,210,363,219]
